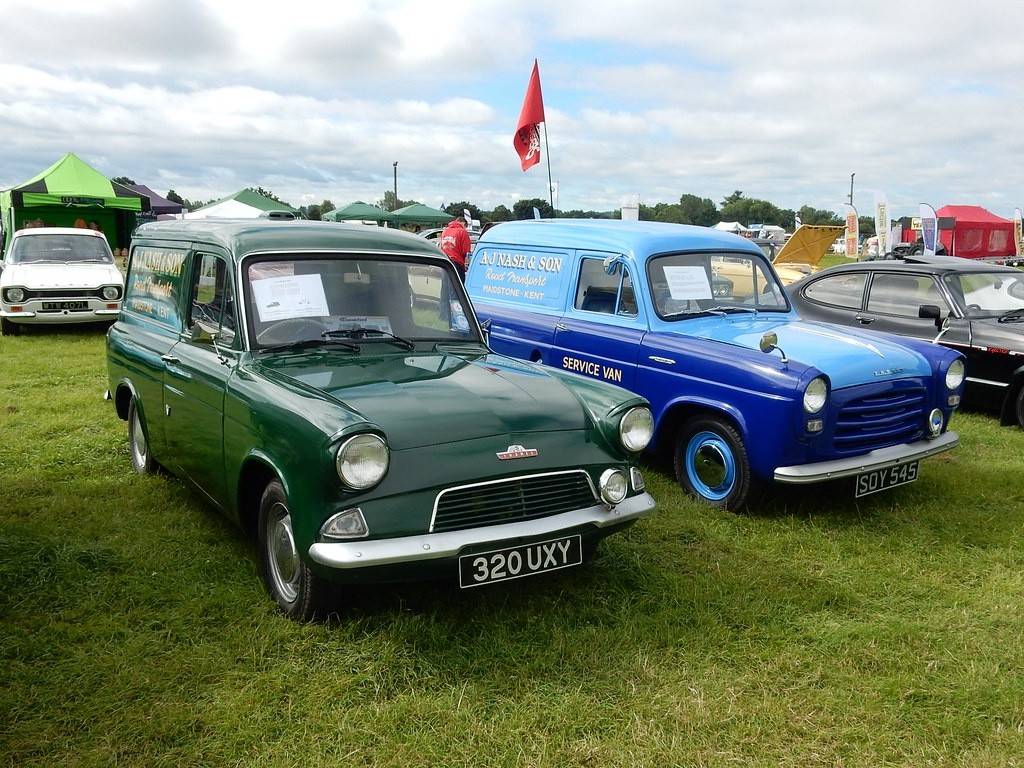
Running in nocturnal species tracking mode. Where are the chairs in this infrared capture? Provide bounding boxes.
[881,278,919,318]
[925,280,951,318]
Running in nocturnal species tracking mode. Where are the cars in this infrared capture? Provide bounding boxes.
[107,210,656,621]
[830,239,846,254]
[450,218,970,517]
[747,237,787,262]
[740,253,1024,431]
[708,253,811,298]
[407,227,482,308]
[0,227,125,334]
[858,235,880,261]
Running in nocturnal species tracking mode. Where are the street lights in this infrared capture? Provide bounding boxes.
[392,161,398,211]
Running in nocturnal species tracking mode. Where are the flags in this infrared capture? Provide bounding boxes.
[514,58,545,172]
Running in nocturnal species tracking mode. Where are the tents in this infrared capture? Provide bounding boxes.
[748,224,786,241]
[709,221,748,231]
[894,205,1016,258]
[0,151,458,262]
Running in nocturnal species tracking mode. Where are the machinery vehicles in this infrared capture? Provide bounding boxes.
[884,236,948,261]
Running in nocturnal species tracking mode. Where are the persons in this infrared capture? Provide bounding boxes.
[439,217,471,320]
[74,217,103,233]
